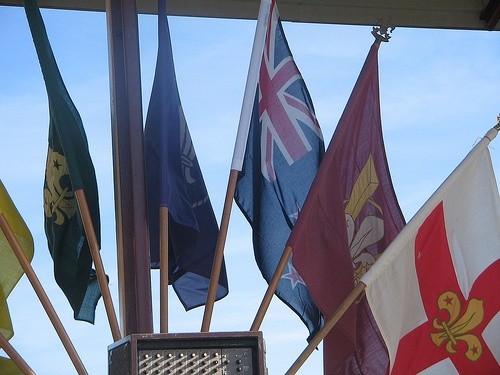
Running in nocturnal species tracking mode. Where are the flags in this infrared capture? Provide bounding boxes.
[287,47,408,375]
[142,0,230,311]
[362,122,500,375]
[0,178,35,350]
[231,0,324,354]
[23,0,110,326]
